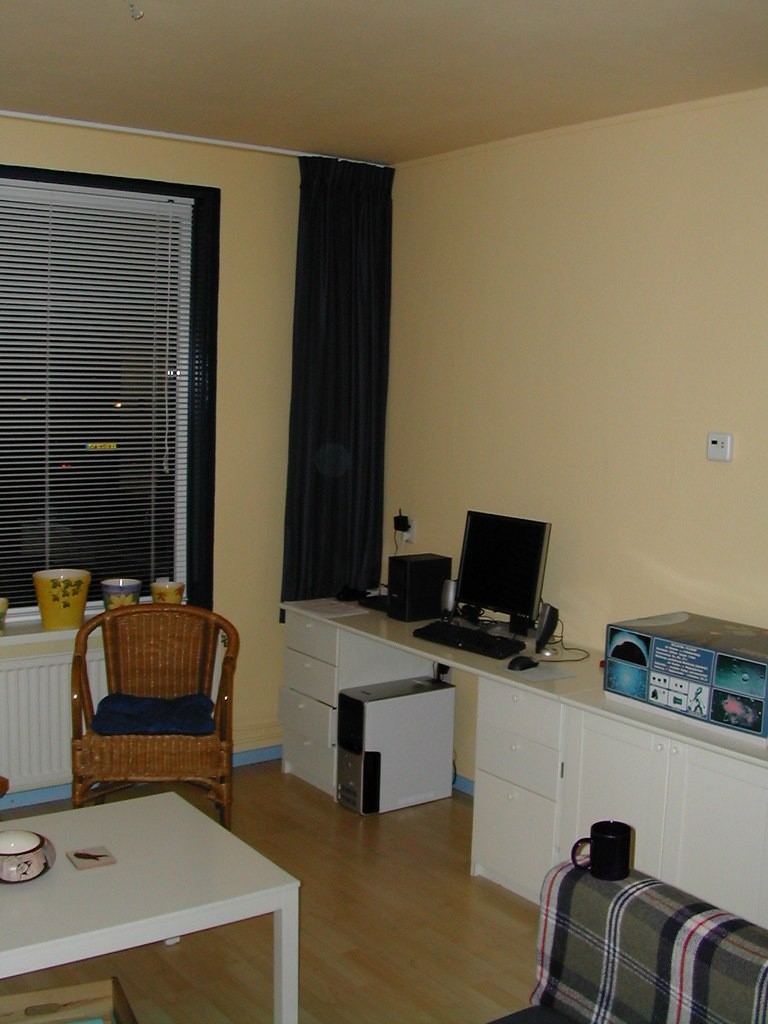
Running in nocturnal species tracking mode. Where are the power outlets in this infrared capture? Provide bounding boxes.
[706,432,733,461]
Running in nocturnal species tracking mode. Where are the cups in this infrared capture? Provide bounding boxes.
[101,579,142,611]
[571,821,633,880]
[0,598,8,631]
[150,581,185,606]
[33,568,92,630]
[0,830,47,884]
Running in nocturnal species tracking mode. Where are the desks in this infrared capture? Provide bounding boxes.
[1,791,300,1024]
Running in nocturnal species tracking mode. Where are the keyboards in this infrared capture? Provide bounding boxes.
[413,620,526,660]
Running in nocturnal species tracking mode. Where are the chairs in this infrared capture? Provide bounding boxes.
[72,604,240,828]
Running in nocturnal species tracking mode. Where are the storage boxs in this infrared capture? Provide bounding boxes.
[605,610,768,747]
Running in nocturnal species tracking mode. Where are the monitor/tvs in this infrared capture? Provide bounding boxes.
[456,511,552,645]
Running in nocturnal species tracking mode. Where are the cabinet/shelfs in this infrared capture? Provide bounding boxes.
[278,598,768,927]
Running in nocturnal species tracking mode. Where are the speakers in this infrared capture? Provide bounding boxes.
[535,604,559,656]
[440,580,456,619]
[386,552,451,622]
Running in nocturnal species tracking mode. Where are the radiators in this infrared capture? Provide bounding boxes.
[0,649,110,792]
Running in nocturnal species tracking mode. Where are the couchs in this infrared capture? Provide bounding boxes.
[487,857,768,1024]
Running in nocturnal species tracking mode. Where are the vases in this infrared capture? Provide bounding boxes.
[102,578,144,611]
[34,569,91,630]
[151,581,187,603]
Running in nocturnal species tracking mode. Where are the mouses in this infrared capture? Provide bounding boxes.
[508,656,539,671]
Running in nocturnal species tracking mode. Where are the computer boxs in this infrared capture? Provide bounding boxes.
[336,676,454,817]
